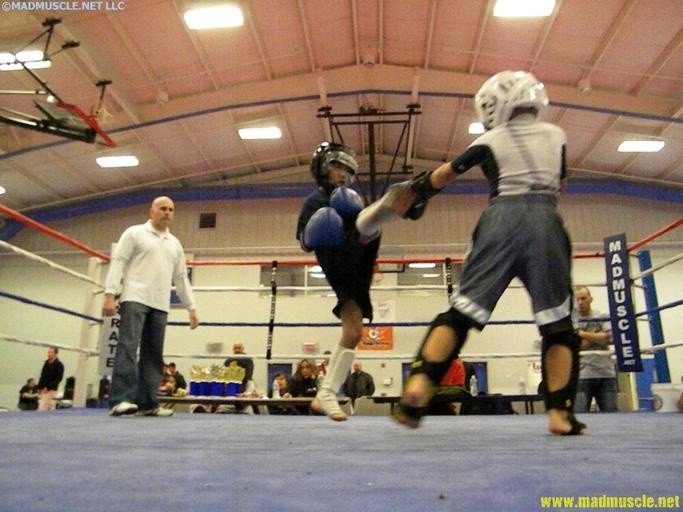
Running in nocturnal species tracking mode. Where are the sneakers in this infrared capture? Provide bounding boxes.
[108,401,137,416]
[144,406,173,416]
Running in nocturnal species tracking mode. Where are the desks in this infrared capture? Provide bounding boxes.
[158,395,350,414]
[366,394,541,414]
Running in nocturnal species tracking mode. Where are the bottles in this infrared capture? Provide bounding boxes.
[518,375,526,395]
[272,377,280,399]
[470,374,478,397]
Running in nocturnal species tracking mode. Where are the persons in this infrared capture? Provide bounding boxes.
[391,70,588,436]
[99,376,110,401]
[159,363,176,394]
[319,350,333,385]
[295,142,417,422]
[36,347,64,411]
[102,196,199,417]
[571,286,620,413]
[344,361,375,397]
[169,363,187,391]
[224,342,252,392]
[18,379,38,410]
[268,371,290,398]
[288,358,319,397]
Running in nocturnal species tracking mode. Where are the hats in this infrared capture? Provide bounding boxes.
[473,70,548,131]
[309,142,357,194]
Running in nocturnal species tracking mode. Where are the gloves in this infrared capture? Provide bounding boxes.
[387,170,444,222]
[329,187,364,215]
[301,206,342,252]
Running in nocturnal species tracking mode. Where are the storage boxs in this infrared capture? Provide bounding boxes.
[189,381,243,396]
